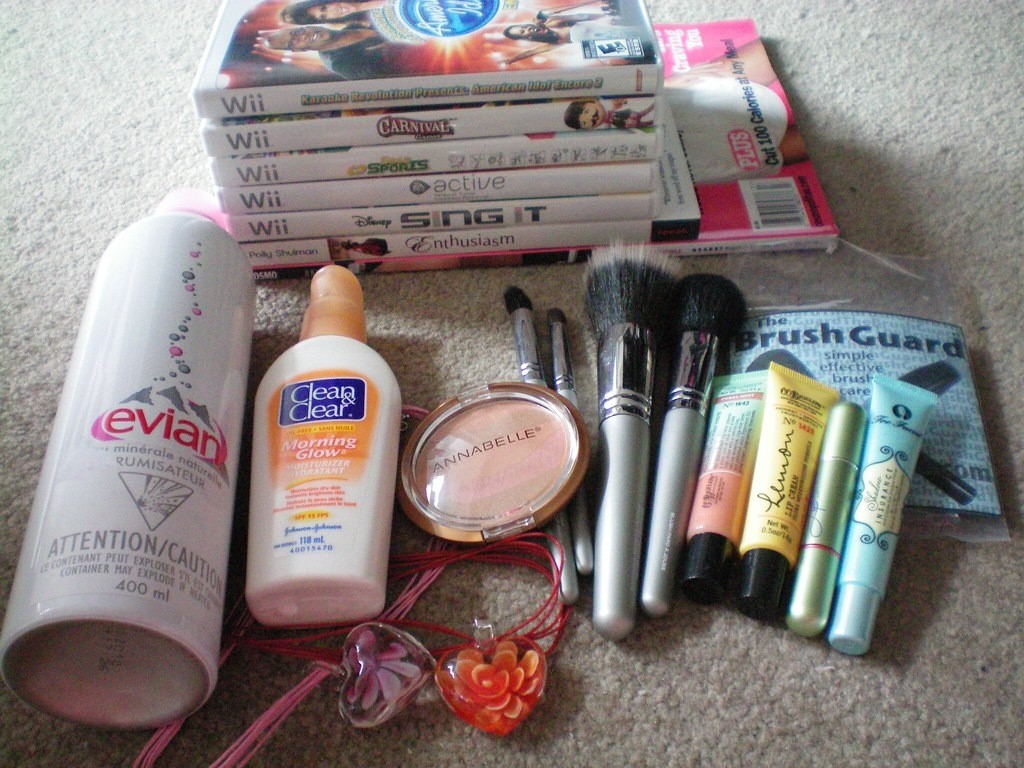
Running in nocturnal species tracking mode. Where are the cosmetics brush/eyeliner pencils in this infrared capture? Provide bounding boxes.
[503,243,747,641]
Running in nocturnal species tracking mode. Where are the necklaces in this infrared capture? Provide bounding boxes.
[132,531,574,768]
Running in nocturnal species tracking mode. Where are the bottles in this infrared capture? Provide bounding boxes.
[0,186,257,732]
[244,265,403,628]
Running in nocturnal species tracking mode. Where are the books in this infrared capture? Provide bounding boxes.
[192,0,841,283]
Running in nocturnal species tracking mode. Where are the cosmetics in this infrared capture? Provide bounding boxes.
[683,362,938,656]
[243,264,401,628]
[399,382,590,544]
[0,185,256,730]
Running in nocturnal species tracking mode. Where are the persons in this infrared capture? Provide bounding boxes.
[291,0,431,44]
[664,37,810,184]
[498,0,624,64]
[265,21,434,80]
